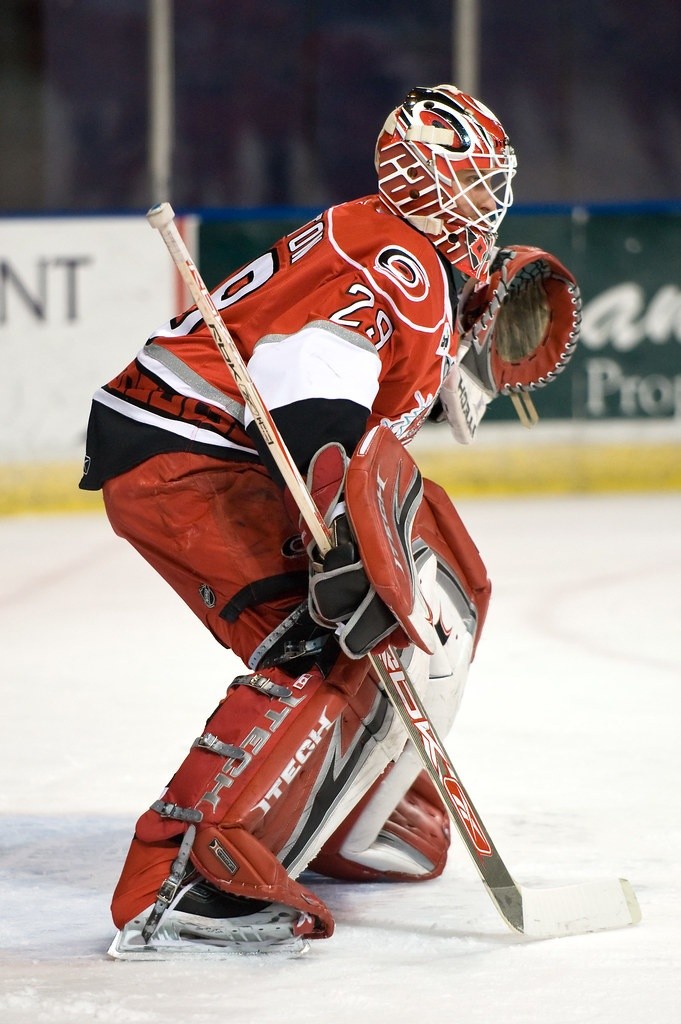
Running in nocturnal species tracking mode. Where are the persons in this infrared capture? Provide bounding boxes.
[76,84,583,959]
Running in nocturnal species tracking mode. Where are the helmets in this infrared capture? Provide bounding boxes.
[374,83,520,285]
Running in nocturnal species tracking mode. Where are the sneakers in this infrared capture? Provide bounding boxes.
[107,857,310,963]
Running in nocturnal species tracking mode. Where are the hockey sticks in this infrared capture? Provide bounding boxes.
[147,201,643,939]
